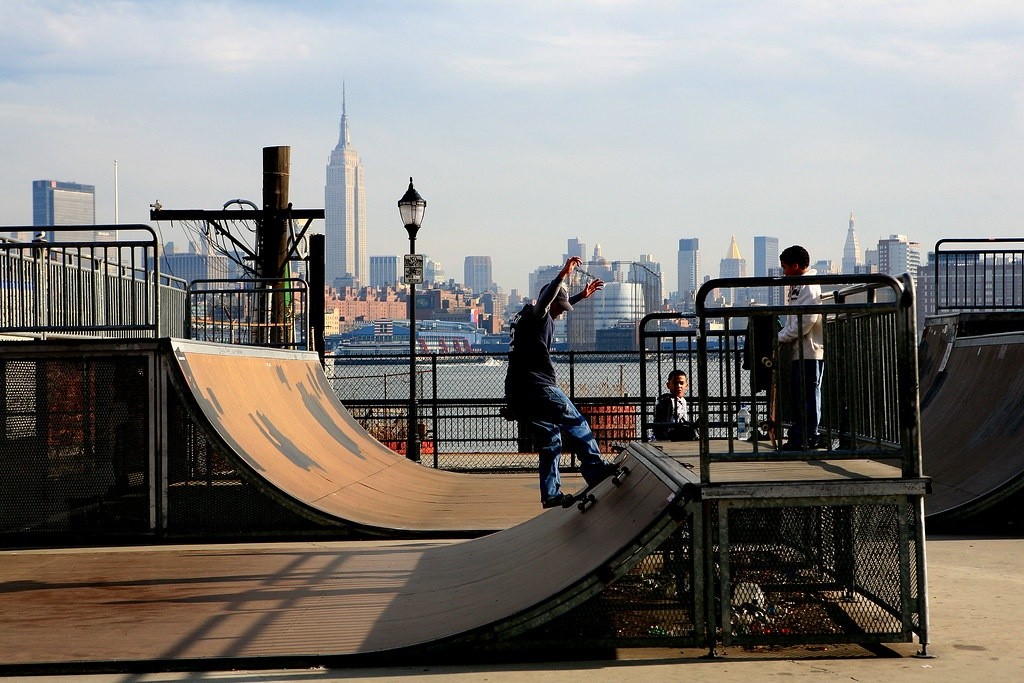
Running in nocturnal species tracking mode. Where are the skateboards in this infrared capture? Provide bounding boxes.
[562,450,631,512]
[759,340,782,451]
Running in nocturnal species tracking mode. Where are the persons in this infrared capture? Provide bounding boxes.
[507,245,825,508]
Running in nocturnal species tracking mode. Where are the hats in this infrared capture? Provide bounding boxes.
[539,282,574,311]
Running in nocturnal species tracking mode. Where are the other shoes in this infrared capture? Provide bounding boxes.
[587,461,618,487]
[775,440,808,451]
[542,493,572,508]
[811,433,829,449]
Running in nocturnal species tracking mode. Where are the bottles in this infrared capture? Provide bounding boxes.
[737,404,750,441]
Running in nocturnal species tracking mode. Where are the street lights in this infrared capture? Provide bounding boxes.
[396,177,428,464]
